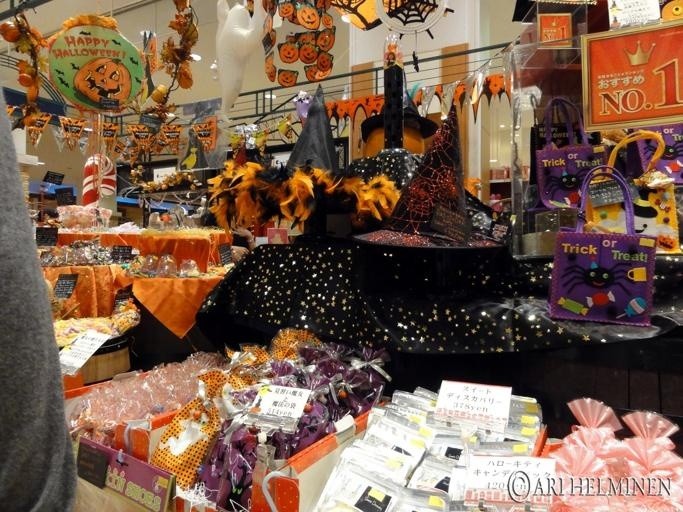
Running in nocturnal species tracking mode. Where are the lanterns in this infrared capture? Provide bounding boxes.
[247,1,336,87]
[72,54,131,109]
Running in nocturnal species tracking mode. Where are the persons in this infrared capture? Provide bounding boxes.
[34,208,61,227]
[199,205,256,263]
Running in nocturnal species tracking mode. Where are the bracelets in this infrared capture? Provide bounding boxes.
[246,235,255,244]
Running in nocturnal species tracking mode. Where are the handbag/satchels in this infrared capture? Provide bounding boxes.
[528,122,602,186]
[627,124,683,184]
[536,144,606,212]
[550,226,657,327]
[586,174,680,255]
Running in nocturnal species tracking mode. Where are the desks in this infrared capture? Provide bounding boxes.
[34,223,236,268]
[34,250,127,341]
[125,268,242,364]
